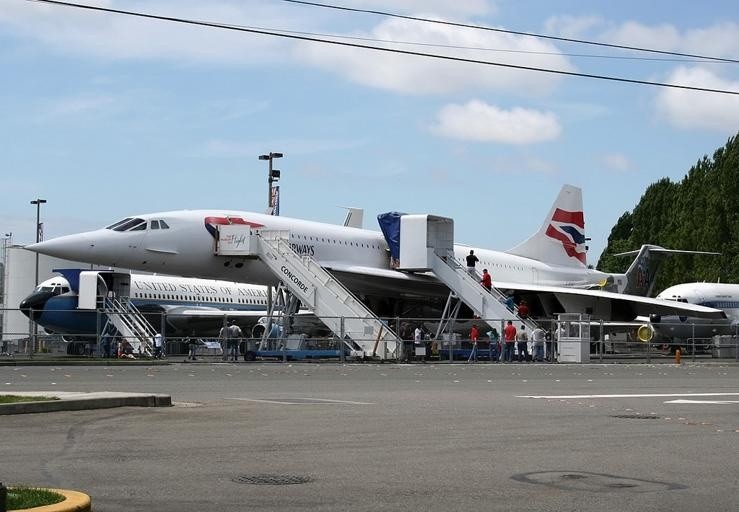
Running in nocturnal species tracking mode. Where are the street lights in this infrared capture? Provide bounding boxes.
[259,152,283,351]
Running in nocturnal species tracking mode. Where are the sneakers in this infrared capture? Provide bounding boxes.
[153,354,238,361]
[409,357,555,363]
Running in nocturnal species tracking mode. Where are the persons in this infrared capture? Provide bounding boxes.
[101,328,167,360]
[498,292,515,314]
[185,329,202,361]
[508,290,514,301]
[388,319,567,363]
[480,278,484,287]
[228,319,245,361]
[217,321,236,360]
[481,269,492,294]
[515,300,529,320]
[523,300,527,306]
[465,250,479,280]
